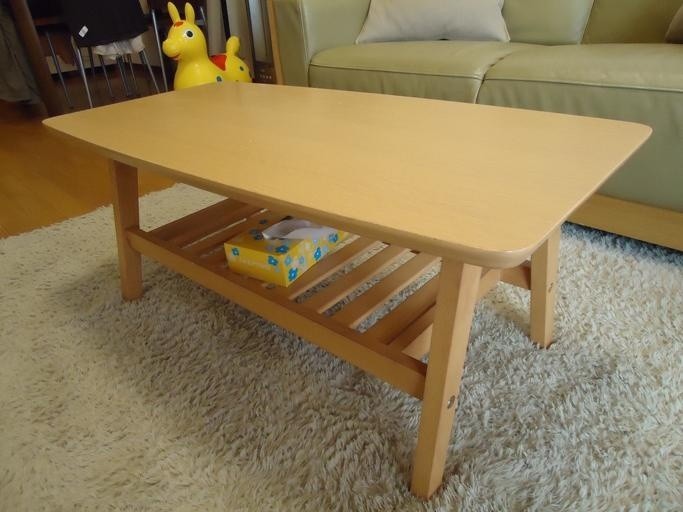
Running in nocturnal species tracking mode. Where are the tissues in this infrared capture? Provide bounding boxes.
[223,209,347,288]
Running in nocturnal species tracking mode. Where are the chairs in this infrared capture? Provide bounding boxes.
[42,1,161,109]
[110,0,207,96]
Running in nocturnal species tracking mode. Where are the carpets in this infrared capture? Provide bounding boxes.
[0,182,683,512]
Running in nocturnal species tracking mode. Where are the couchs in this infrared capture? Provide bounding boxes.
[271,0,683,254]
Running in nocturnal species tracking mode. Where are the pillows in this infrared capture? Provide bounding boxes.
[662,3,683,44]
[355,0,511,44]
